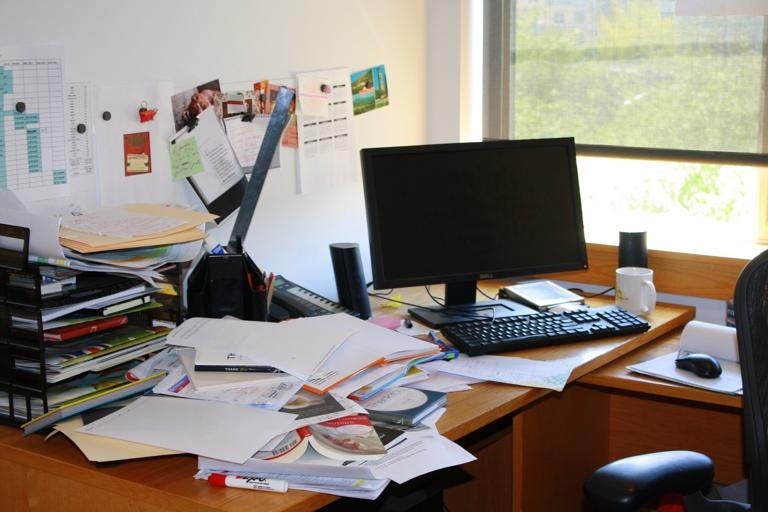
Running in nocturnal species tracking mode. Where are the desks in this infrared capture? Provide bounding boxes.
[0,242,750,512]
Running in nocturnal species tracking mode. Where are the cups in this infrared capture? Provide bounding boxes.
[614,266,658,320]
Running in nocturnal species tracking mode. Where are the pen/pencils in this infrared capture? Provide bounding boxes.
[405,318,413,328]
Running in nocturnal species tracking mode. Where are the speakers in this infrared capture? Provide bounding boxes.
[329,242,371,318]
[618,231,648,269]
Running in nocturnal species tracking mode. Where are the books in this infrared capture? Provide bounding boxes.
[1,202,456,501]
[503,280,584,313]
[624,317,744,396]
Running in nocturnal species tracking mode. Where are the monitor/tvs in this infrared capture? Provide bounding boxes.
[360,136,589,329]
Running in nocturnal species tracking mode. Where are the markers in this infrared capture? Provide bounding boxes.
[208,472,288,493]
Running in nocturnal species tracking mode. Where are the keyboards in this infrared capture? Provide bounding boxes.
[440,305,652,357]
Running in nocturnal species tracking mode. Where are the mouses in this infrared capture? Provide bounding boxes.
[675,352,722,379]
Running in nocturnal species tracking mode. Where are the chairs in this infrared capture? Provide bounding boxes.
[582,249,766,511]
